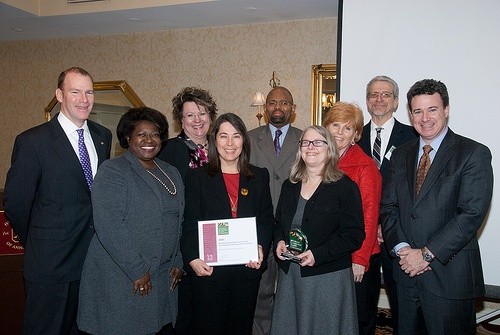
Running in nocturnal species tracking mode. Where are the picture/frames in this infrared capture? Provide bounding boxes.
[311,64,336,126]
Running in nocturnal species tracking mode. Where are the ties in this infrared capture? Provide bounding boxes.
[274,130,283,154]
[76,129,94,191]
[373,128,384,169]
[416,146,433,198]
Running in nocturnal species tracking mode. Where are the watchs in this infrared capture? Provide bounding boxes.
[422,246,433,263]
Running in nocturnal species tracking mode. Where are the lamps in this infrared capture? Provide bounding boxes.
[251,91,266,126]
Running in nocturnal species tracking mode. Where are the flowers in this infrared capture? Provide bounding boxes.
[189,147,209,168]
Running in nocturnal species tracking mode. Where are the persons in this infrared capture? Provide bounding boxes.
[155,83,219,176]
[320,101,381,335]
[181,113,275,335]
[4,65,112,335]
[271,125,366,335]
[77,106,186,335]
[245,87,304,335]
[353,75,419,334]
[381,78,494,335]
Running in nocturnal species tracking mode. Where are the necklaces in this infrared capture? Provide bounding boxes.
[145,161,176,195]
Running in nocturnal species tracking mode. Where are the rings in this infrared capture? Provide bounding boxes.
[140,287,144,291]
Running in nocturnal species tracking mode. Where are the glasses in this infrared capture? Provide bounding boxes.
[369,92,393,98]
[298,140,328,147]
